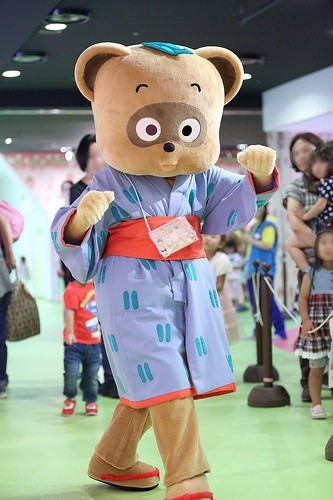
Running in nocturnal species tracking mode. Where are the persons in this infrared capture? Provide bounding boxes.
[51,133,121,400]
[282,131,333,402]
[203,201,287,343]
[299,230,333,419]
[291,144,333,231]
[157,240,167,254]
[62,278,102,415]
[0,214,16,398]
[18,256,30,284]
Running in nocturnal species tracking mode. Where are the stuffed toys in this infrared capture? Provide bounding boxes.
[51,43,280,500]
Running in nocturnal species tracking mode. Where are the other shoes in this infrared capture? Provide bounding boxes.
[0,381,6,398]
[301,387,311,403]
[61,399,75,416]
[311,404,325,418]
[85,402,97,415]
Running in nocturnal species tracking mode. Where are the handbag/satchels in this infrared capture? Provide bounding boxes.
[4,266,40,342]
